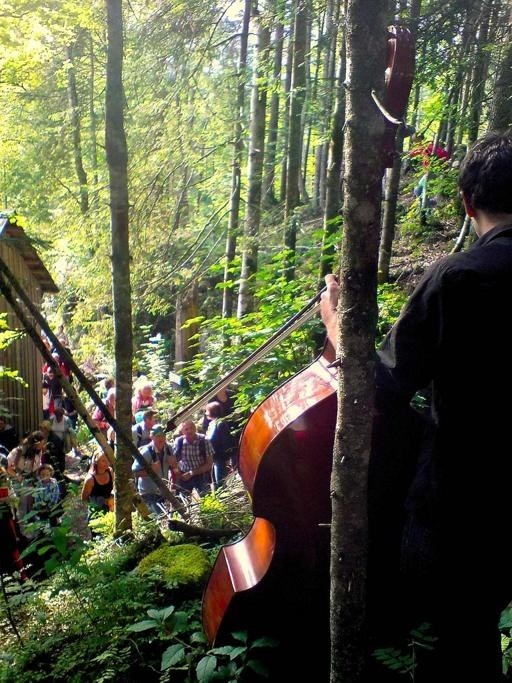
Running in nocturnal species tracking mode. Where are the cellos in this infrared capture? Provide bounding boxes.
[201,23,425,651]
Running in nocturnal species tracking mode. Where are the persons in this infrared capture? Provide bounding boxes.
[320,127,511,681]
[1,323,255,591]
